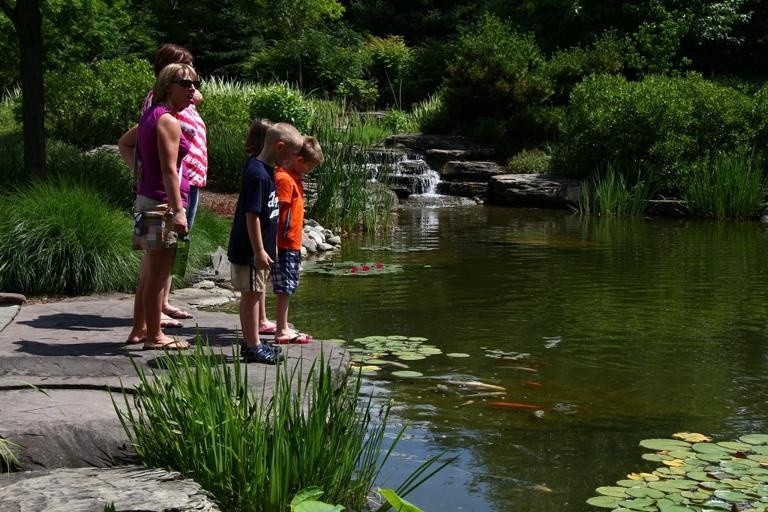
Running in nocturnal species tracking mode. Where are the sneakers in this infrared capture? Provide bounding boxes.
[257,319,278,335]
[239,338,282,356]
[242,343,285,366]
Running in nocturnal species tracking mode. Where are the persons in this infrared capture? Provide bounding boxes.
[226,121,303,365]
[273,134,326,345]
[143,43,209,329]
[244,118,278,336]
[117,61,202,351]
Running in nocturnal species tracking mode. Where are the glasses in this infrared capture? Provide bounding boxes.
[168,78,201,90]
[132,211,145,237]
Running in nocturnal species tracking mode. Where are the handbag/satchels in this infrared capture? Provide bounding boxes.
[131,209,178,252]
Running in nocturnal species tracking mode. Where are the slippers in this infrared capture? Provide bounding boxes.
[160,313,183,328]
[142,337,191,351]
[275,327,314,345]
[166,308,194,320]
[124,336,147,344]
